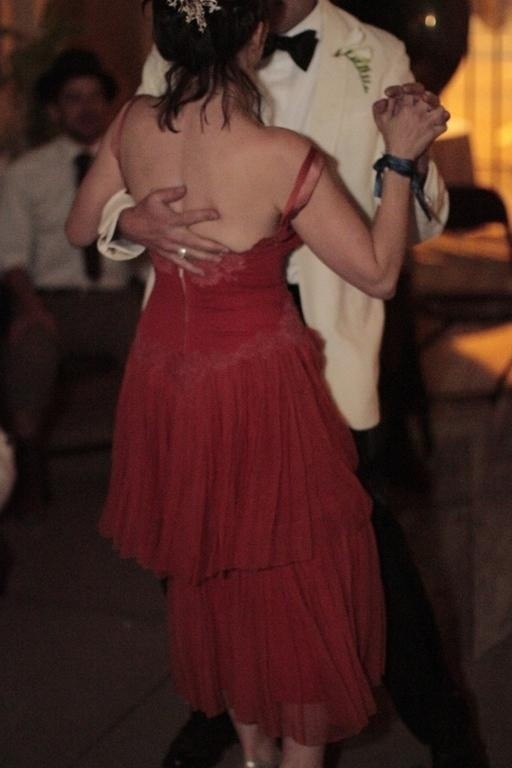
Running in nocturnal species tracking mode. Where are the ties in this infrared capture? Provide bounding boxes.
[72,148,102,281]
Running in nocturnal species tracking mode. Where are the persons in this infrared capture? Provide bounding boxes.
[98,0,506,764]
[0,46,150,453]
[65,1,449,765]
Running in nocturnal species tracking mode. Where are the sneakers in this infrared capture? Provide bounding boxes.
[160,705,239,768]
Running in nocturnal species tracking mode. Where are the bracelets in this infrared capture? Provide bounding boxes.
[371,151,438,222]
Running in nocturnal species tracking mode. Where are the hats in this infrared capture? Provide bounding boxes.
[33,49,117,102]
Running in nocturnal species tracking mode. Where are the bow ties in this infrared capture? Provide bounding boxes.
[261,29,319,71]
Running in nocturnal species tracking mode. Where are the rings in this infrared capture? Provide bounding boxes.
[177,243,189,262]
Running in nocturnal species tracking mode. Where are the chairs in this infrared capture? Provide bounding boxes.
[388,133,512,455]
